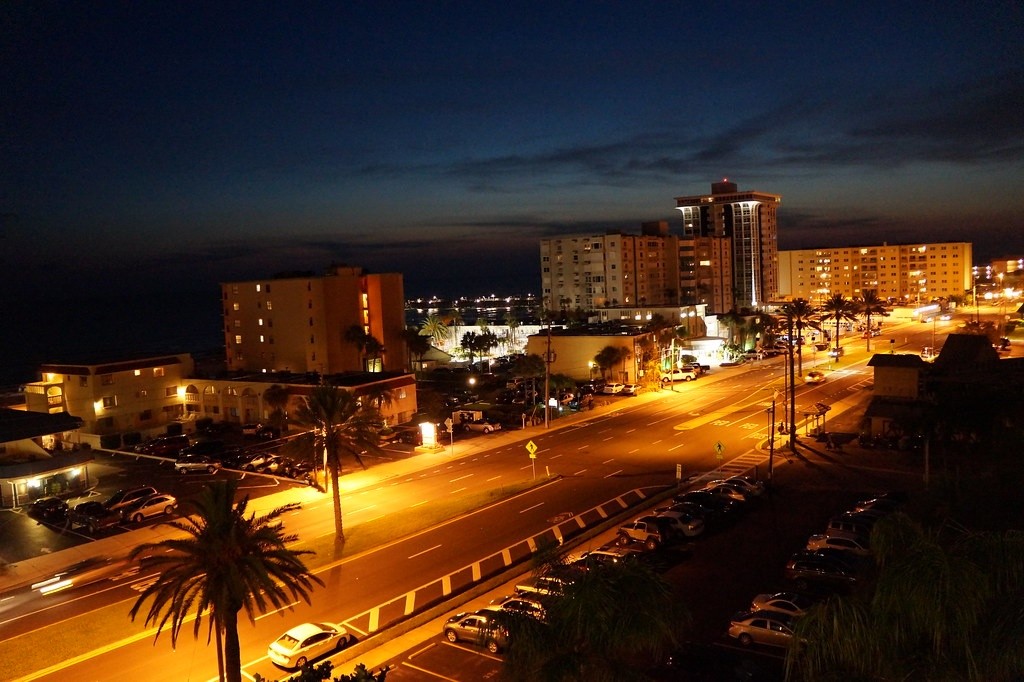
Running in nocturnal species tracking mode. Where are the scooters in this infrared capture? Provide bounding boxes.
[856,432,924,451]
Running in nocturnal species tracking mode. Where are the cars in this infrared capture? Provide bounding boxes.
[741,333,805,359]
[992,338,1012,351]
[478,484,753,633]
[728,610,815,650]
[603,382,625,395]
[63,501,124,535]
[661,362,711,384]
[846,322,882,339]
[378,420,462,448]
[750,593,813,621]
[102,484,159,513]
[132,418,314,479]
[442,610,513,653]
[621,383,642,396]
[27,497,69,520]
[707,475,768,498]
[267,621,351,670]
[461,417,502,435]
[830,346,844,358]
[413,352,605,413]
[121,493,179,523]
[804,371,826,384]
[781,487,906,594]
